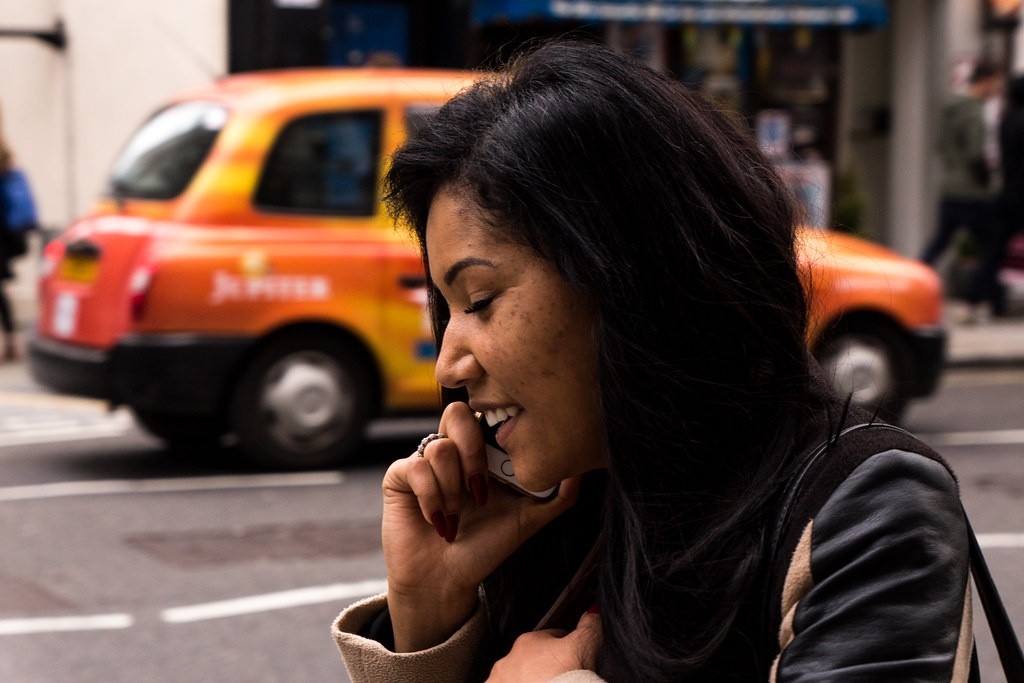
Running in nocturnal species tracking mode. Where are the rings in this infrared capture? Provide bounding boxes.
[416,433,449,458]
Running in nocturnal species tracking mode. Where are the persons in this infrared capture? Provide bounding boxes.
[0,135,40,363]
[331,37,982,683]
[917,58,1024,320]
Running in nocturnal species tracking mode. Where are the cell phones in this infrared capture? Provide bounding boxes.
[474,410,561,504]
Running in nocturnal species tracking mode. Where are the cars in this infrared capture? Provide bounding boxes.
[23,69,950,473]
